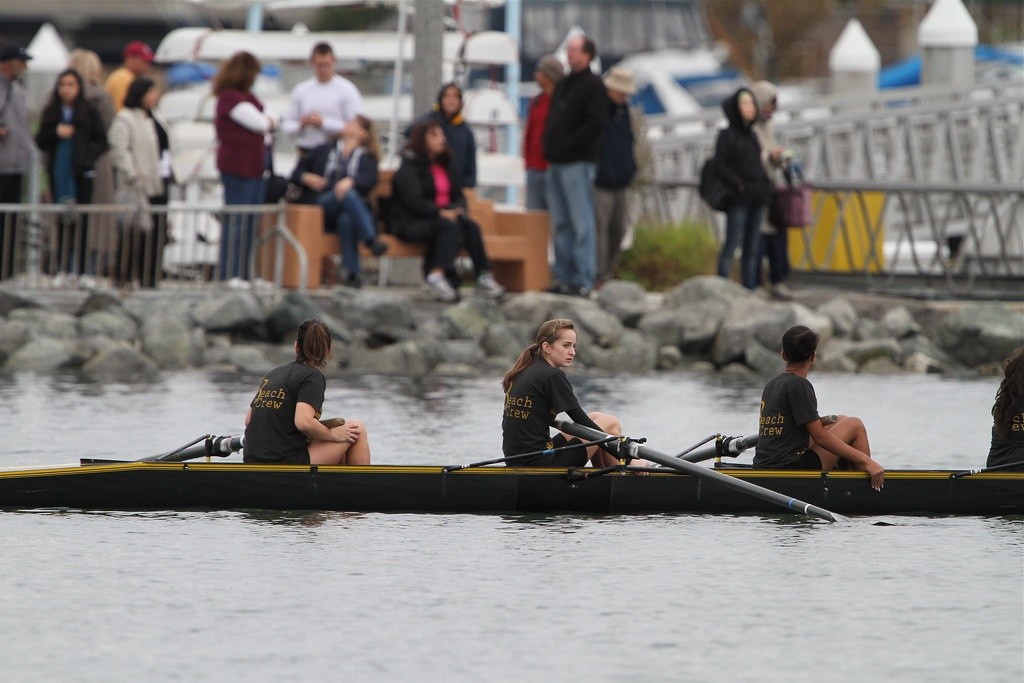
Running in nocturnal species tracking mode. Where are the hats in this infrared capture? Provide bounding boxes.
[125,41,154,62]
[0,43,32,61]
[603,66,638,95]
[536,54,565,84]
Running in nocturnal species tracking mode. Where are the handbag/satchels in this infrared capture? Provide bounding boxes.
[700,128,741,211]
[110,174,152,231]
[770,155,810,228]
[265,147,287,203]
[616,189,640,250]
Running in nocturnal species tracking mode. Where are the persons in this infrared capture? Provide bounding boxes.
[714,88,772,290]
[751,81,795,298]
[593,67,638,282]
[986,347,1024,473]
[242,318,370,465]
[283,43,367,161]
[290,113,389,288]
[500,319,650,476]
[523,55,565,210]
[401,82,476,222]
[542,36,607,297]
[384,120,506,303]
[210,52,282,290]
[753,325,884,491]
[0,41,174,292]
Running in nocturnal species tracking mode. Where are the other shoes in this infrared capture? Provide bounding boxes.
[51,270,67,289]
[369,240,389,257]
[254,277,272,289]
[421,273,455,304]
[540,283,589,298]
[345,274,361,289]
[475,273,504,297]
[79,274,95,288]
[771,282,794,301]
[230,277,249,289]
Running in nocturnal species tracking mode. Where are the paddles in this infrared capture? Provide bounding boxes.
[552,418,850,524]
[80,417,347,465]
[653,414,840,468]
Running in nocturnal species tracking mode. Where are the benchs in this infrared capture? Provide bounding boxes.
[259,170,549,292]
